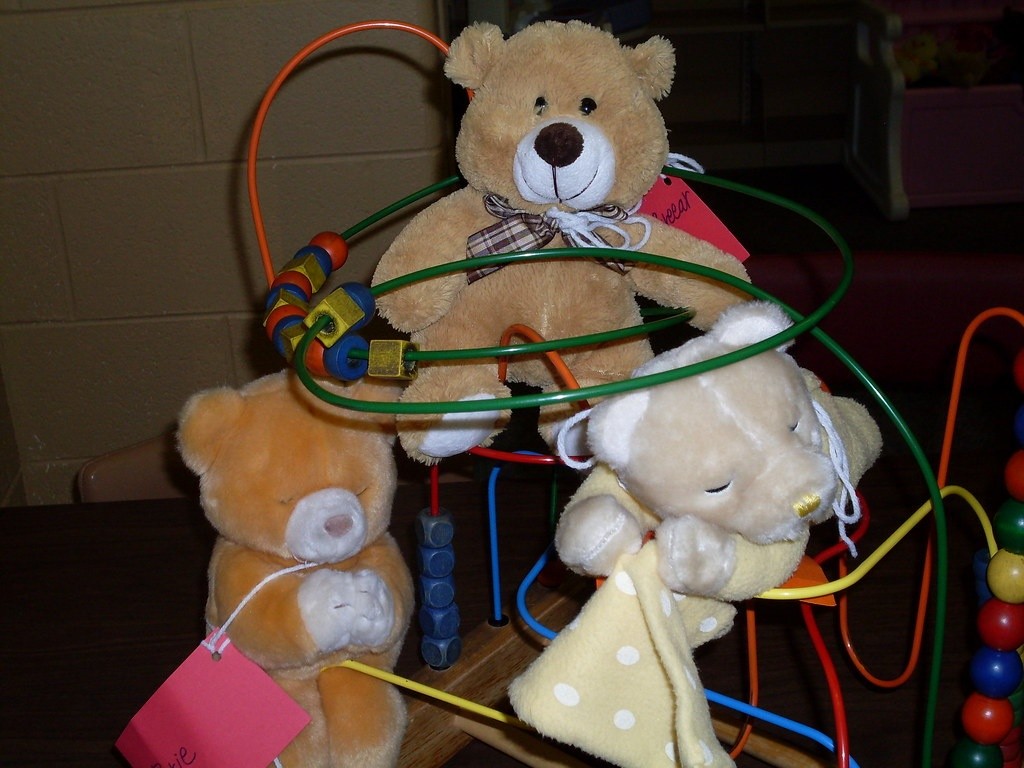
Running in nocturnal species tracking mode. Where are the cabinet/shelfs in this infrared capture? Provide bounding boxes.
[468,0,855,169]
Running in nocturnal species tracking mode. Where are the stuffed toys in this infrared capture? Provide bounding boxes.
[371,20,756,468]
[553,301,882,602]
[175,368,417,768]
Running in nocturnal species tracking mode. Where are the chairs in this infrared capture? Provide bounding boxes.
[841,0,1024,221]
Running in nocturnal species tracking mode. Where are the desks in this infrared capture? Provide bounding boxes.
[0,442,1024,768]
